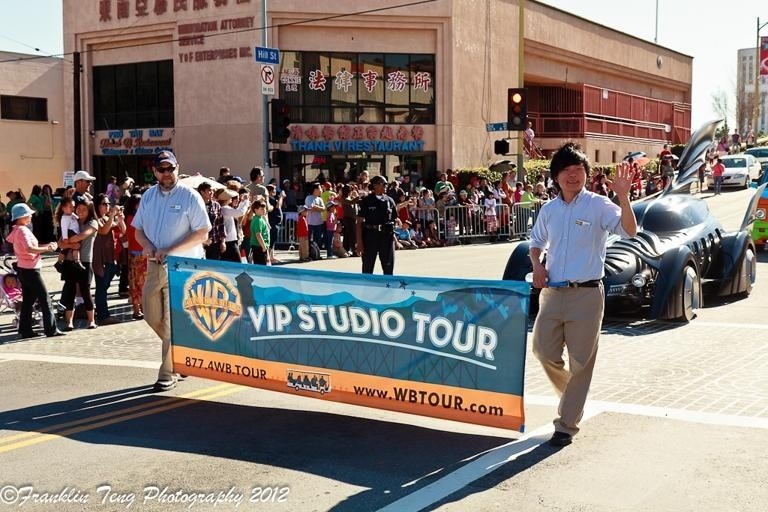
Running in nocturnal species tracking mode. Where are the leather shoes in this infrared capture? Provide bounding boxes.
[21,331,42,338]
[550,432,573,446]
[155,380,177,390]
[47,332,67,336]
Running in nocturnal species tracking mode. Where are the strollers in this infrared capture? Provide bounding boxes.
[0,256,43,328]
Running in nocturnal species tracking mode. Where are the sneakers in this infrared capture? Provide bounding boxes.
[88,325,97,330]
[133,313,146,320]
[100,316,119,325]
[300,257,313,263]
[60,327,75,332]
[327,254,337,258]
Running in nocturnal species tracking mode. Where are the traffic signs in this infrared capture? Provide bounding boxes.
[254,46,280,65]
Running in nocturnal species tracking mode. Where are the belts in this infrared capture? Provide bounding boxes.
[150,259,166,264]
[567,280,604,289]
[367,224,391,231]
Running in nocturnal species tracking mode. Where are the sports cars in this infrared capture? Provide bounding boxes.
[502,117,768,330]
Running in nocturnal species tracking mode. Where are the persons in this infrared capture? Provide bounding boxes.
[296,172,367,261]
[0,189,26,256]
[2,273,24,315]
[590,123,754,209]
[199,166,295,265]
[40,185,53,243]
[358,175,400,276]
[73,170,97,318]
[393,168,558,250]
[129,149,213,391]
[27,184,45,244]
[6,203,67,338]
[52,187,75,310]
[92,193,127,325]
[524,121,535,147]
[106,176,121,207]
[529,144,637,446]
[125,193,148,319]
[54,198,86,273]
[57,198,98,330]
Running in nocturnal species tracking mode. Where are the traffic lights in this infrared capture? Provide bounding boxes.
[270,98,292,145]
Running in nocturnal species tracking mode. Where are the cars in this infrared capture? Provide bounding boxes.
[753,163,767,251]
[707,153,762,190]
[743,146,768,169]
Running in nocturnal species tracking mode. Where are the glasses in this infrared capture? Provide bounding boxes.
[379,182,388,185]
[102,202,111,206]
[156,165,176,172]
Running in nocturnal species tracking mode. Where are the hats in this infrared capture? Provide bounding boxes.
[233,177,246,184]
[11,203,36,221]
[214,189,238,201]
[298,205,312,213]
[326,201,338,209]
[460,190,468,195]
[324,181,332,189]
[155,151,177,167]
[283,179,289,183]
[73,170,96,181]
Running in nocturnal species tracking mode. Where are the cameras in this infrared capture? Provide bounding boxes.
[119,206,125,212]
